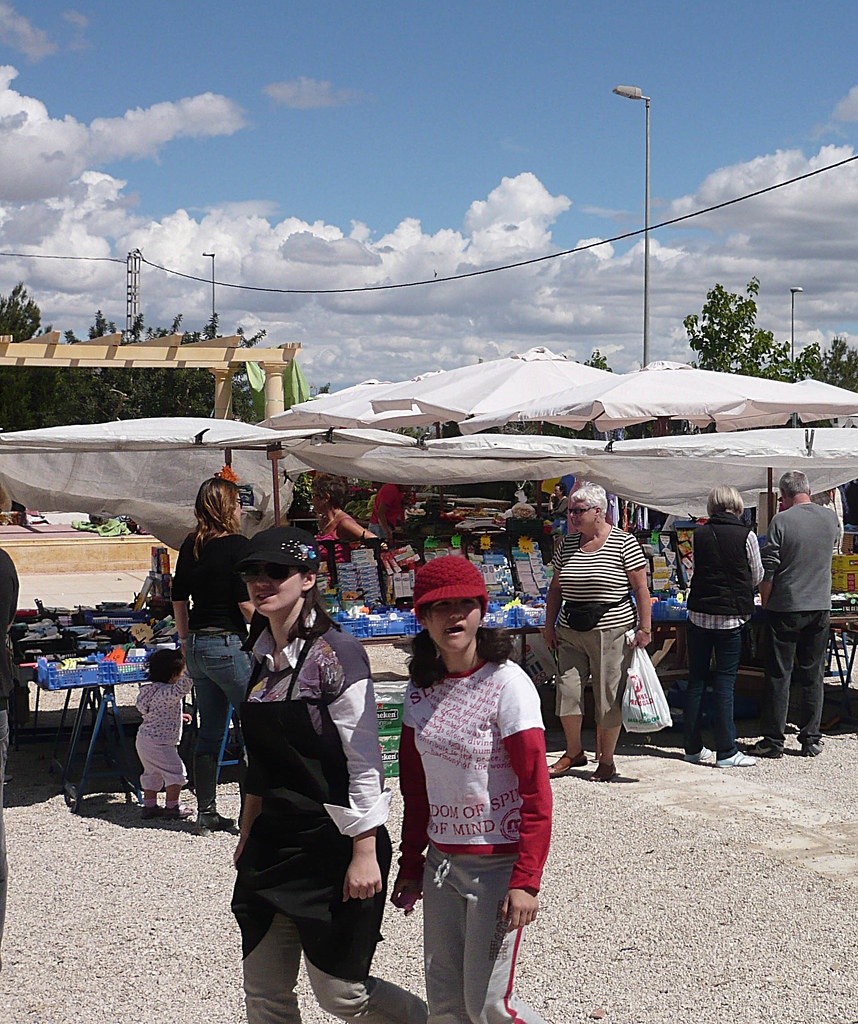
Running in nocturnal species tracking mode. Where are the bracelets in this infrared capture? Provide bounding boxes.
[640,626,651,634]
[179,637,188,645]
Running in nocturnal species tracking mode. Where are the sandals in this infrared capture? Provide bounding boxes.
[588,763,615,782]
[547,749,588,776]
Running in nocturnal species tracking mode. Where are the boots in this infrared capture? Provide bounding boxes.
[192,752,236,832]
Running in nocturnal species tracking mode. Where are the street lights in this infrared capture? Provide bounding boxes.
[613,84,652,438]
[201,251,216,319]
[789,285,803,364]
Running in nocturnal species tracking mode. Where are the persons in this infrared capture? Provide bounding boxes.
[0,546,20,976]
[135,478,552,1024]
[311,474,839,781]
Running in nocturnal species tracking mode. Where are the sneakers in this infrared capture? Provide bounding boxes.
[684,746,716,764]
[714,751,757,769]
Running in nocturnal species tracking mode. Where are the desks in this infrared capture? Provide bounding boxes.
[356,624,545,674]
[648,619,690,681]
[27,679,247,815]
[824,613,858,730]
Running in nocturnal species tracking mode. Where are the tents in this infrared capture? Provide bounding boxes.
[0,348,858,552]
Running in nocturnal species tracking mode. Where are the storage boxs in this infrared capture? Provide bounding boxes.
[16,638,78,663]
[832,570,858,594]
[832,553,858,571]
[467,542,548,629]
[151,547,167,574]
[0,511,22,525]
[84,609,151,624]
[96,649,153,682]
[160,553,170,575]
[150,571,156,596]
[146,600,190,621]
[37,653,99,690]
[378,735,400,777]
[155,571,172,600]
[375,702,404,736]
[14,613,42,624]
[735,670,766,702]
[317,544,423,638]
[629,521,697,620]
[506,517,544,536]
[25,510,92,526]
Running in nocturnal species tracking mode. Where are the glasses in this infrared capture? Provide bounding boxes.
[237,563,307,585]
[235,497,244,509]
[567,505,597,516]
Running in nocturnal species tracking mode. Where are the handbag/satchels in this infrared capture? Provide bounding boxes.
[744,621,758,659]
[621,630,674,733]
[562,595,630,633]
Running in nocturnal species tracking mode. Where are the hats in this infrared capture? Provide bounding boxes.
[412,555,489,624]
[238,526,321,577]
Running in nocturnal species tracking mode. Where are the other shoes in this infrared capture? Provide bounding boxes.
[163,804,194,820]
[742,741,783,758]
[4,774,14,783]
[140,804,166,820]
[802,739,826,757]
